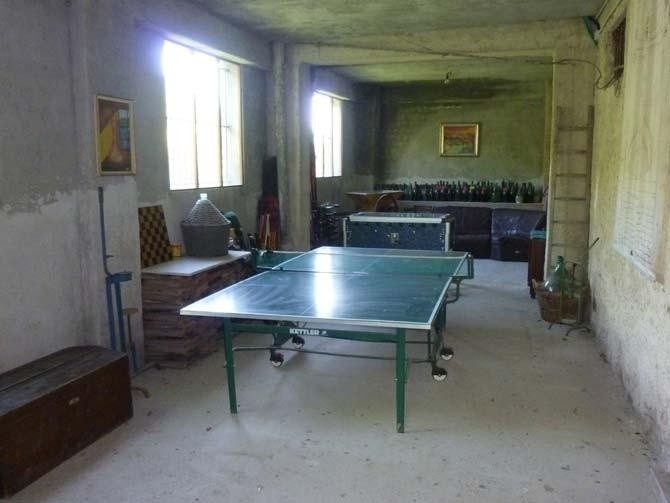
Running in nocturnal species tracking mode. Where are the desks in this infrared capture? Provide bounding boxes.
[179,243,477,435]
[344,190,404,211]
[142,249,252,369]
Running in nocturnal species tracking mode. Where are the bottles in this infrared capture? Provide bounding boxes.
[228,226,241,249]
[377,175,541,203]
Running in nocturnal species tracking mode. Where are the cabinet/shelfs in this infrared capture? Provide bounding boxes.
[434,207,547,262]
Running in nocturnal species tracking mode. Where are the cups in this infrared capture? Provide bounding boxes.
[170,241,182,257]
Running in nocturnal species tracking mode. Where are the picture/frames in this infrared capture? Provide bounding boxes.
[93,93,137,176]
[439,121,481,157]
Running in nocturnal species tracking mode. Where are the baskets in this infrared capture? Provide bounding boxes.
[530,278,588,324]
[180,199,232,257]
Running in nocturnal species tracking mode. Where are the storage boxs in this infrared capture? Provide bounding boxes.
[342,210,456,251]
[0,343,136,501]
